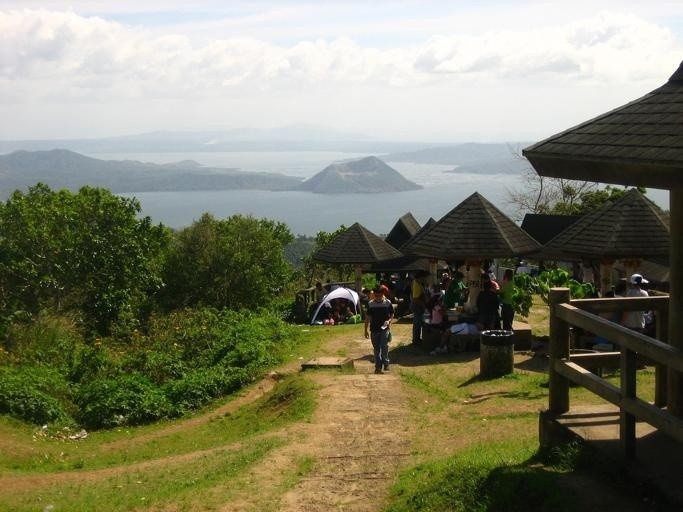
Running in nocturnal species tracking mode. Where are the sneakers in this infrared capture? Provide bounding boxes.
[429,346,449,354]
[375,365,390,373]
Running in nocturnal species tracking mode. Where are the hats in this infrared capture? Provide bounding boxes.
[631,273,650,285]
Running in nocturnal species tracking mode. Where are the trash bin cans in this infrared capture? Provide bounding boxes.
[480,330,514,381]
